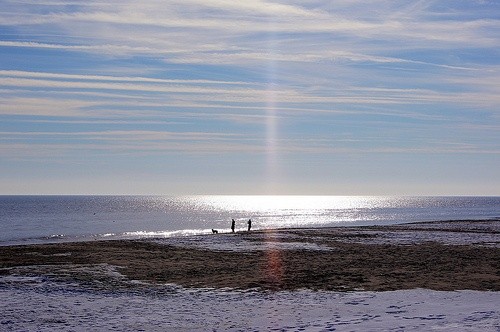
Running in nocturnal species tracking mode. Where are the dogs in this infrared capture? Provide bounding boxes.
[211,229,218,234]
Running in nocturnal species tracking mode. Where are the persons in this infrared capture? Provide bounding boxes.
[231,219,236,234]
[248,219,253,232]
